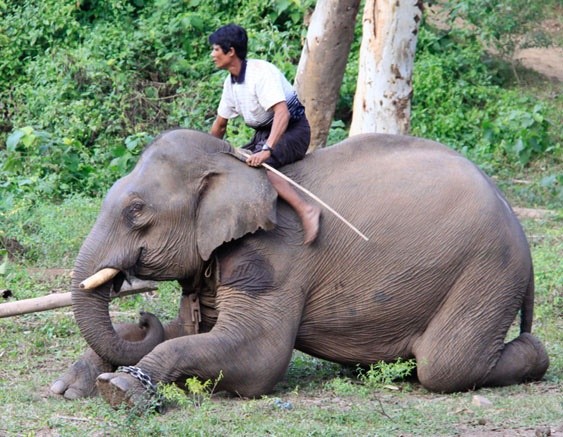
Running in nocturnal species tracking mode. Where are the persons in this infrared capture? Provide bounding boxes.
[208,25,323,244]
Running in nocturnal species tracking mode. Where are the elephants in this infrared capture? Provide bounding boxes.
[51,128,550,419]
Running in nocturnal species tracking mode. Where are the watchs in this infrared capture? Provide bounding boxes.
[262,144,273,156]
